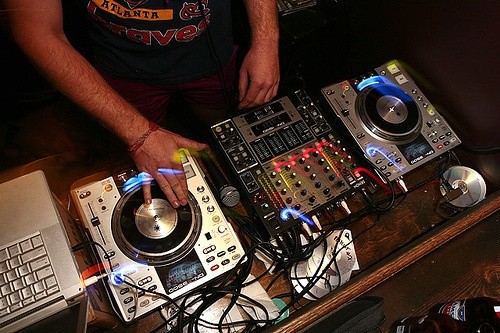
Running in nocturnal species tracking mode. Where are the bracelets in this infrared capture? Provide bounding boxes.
[128,120,159,153]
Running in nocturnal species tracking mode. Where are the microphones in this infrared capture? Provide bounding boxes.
[198,149,240,208]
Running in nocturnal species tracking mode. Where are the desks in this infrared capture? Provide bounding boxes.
[0,95,499,333]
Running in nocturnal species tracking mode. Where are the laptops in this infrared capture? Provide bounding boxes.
[0,170,89,333]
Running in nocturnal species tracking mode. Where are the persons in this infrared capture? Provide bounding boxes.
[8,0,281,209]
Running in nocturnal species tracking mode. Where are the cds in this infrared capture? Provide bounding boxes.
[289,231,356,301]
[439,164,487,208]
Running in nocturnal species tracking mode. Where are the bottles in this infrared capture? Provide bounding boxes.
[430,297,500,333]
[389,314,483,333]
